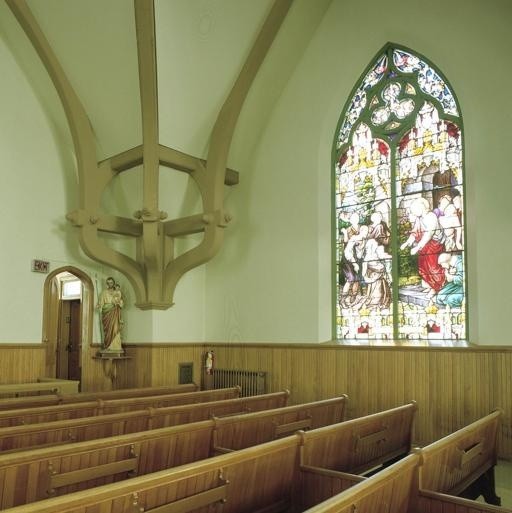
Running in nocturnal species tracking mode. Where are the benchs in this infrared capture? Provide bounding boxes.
[0,383,512,513]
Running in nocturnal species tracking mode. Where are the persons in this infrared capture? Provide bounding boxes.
[113,283,123,307]
[331,46,466,341]
[96,274,126,355]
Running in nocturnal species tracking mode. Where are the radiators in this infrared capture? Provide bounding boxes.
[212,368,266,398]
